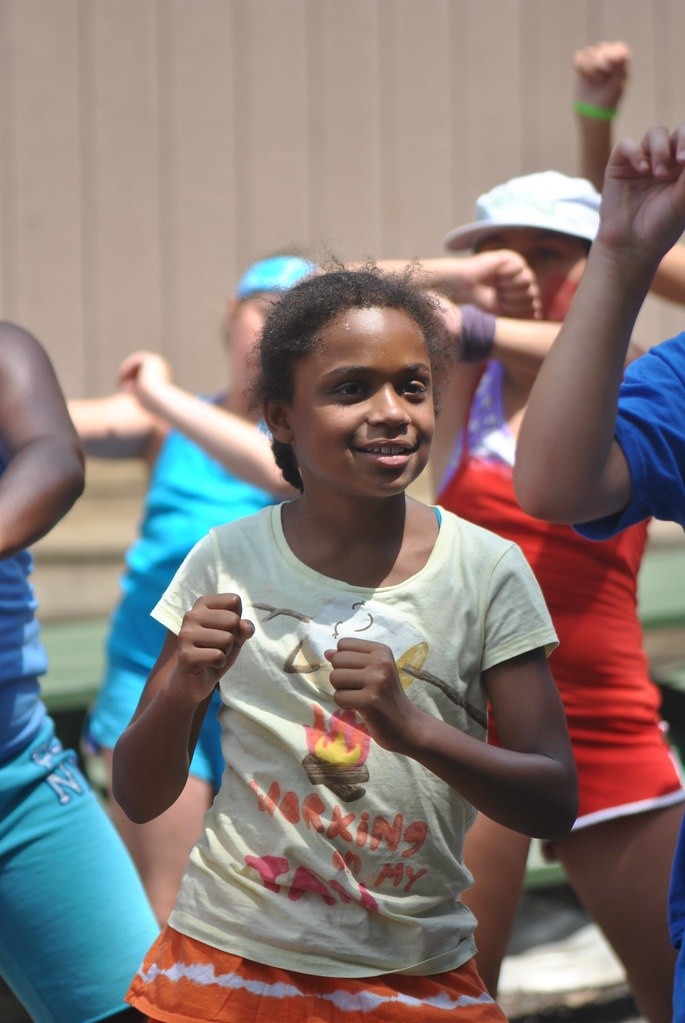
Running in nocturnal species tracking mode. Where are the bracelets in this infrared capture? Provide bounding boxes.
[459,303,496,363]
[572,96,619,123]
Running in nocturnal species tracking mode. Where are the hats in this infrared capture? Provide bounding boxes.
[443,172,601,246]
[238,255,314,295]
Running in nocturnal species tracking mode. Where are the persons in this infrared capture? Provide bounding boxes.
[0,319,164,1023]
[571,38,685,306]
[62,258,334,934]
[329,167,685,1023]
[507,123,685,1023]
[107,271,579,1023]
